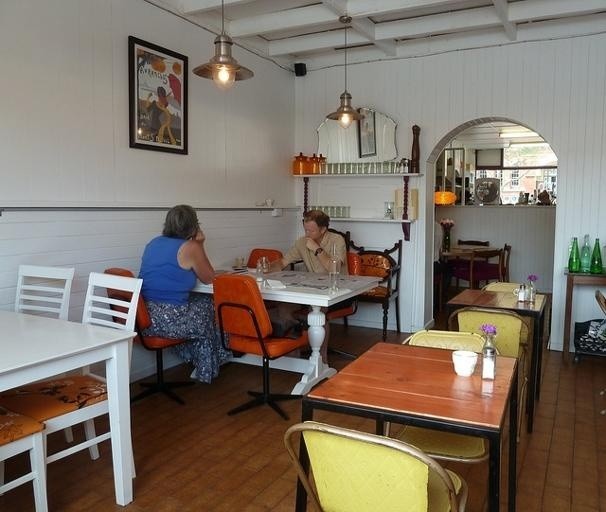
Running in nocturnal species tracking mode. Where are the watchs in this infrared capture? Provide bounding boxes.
[314,247,323,257]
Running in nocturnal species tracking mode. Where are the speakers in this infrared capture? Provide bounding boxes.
[294,62,306,76]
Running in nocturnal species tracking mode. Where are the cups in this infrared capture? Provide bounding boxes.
[453,351,479,378]
[322,160,410,174]
[307,206,350,217]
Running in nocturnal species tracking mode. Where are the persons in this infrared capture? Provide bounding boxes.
[137,204,233,386]
[434,221,445,281]
[144,87,178,146]
[255,209,350,308]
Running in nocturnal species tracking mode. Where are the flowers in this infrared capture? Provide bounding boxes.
[478,324,497,341]
[527,274,537,285]
[441,219,454,229]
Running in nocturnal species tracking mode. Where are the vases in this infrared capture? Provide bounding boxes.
[443,228,450,249]
[527,283,536,301]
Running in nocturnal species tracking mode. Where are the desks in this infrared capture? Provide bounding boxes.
[294,340,519,512]
[288,172,422,240]
[0,308,138,508]
[444,287,547,433]
[561,267,605,364]
[187,266,383,398]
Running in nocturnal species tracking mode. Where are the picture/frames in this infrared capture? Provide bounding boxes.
[125,33,188,155]
[357,108,376,159]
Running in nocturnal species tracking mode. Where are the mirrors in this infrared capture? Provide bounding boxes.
[314,106,398,162]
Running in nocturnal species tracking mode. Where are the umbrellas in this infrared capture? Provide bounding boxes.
[167,73,181,106]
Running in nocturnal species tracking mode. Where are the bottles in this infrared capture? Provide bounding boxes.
[479,346,496,379]
[327,258,341,294]
[517,284,535,303]
[233,255,271,282]
[568,234,604,274]
[291,151,327,175]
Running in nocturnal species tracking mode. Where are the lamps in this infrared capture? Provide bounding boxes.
[189,2,253,87]
[325,15,365,128]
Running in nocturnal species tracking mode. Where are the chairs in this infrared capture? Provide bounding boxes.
[102,266,195,406]
[245,249,284,310]
[0,407,54,512]
[480,281,520,292]
[2,265,75,406]
[209,276,302,422]
[275,420,470,512]
[435,239,511,317]
[444,309,529,446]
[11,272,145,474]
[385,331,498,466]
[291,254,364,359]
[349,241,402,343]
[290,229,358,362]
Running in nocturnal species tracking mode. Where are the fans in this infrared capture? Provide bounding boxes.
[471,177,500,206]
